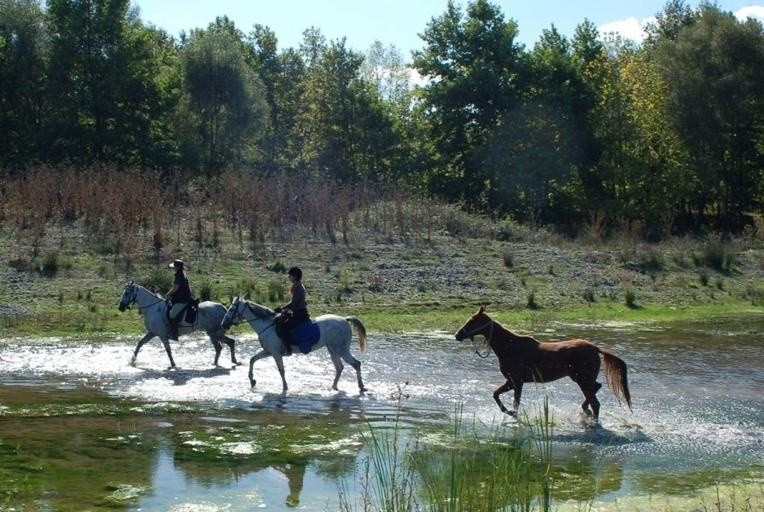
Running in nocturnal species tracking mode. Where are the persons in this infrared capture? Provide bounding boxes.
[164,258,193,342]
[274,267,309,355]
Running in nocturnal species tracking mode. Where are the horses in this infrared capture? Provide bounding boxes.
[118,280,241,368]
[454,305,633,422]
[219,292,368,391]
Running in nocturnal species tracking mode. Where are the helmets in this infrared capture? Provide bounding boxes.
[287,267,302,281]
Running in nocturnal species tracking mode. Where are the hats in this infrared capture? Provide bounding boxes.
[168,259,186,269]
